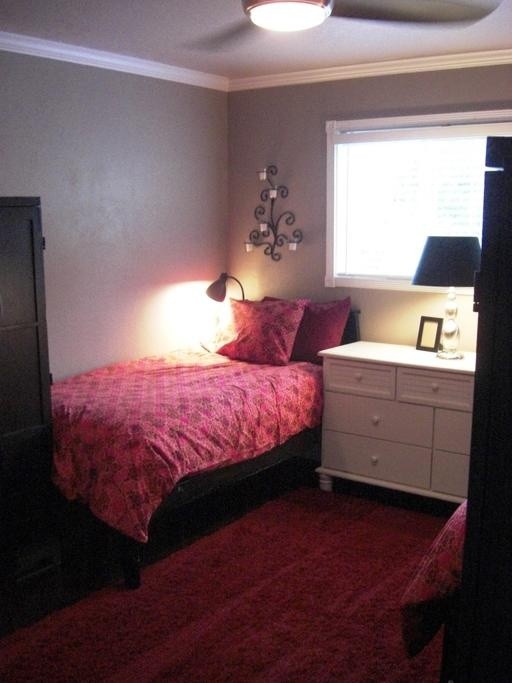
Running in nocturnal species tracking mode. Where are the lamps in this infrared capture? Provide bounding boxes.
[240,0,333,33]
[411,237,480,360]
[206,273,245,302]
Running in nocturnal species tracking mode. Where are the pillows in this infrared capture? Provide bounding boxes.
[263,295,352,364]
[216,300,306,365]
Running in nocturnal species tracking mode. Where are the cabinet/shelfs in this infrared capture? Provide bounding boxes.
[0,196,62,635]
[313,339,475,505]
[451,136,512,682]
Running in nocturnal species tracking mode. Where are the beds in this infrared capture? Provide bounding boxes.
[52,309,362,590]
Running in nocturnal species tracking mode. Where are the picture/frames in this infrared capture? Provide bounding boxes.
[415,316,444,353]
